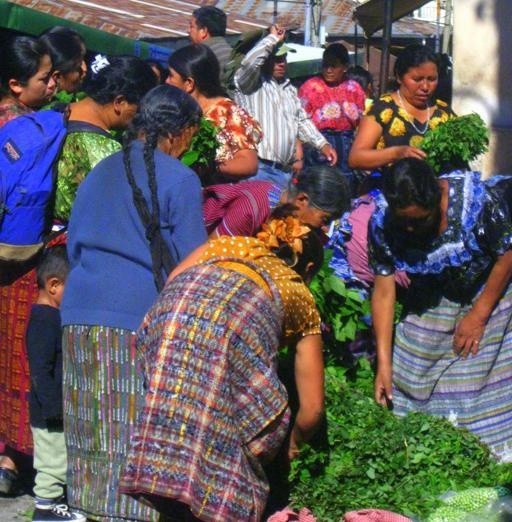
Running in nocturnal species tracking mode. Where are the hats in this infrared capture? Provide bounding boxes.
[272,39,296,58]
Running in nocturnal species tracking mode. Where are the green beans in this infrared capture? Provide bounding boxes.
[446,487,497,513]
[428,507,467,522]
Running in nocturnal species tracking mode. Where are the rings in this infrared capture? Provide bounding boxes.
[473,339,480,345]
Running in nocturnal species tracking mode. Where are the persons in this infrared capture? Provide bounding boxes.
[1,3,460,245]
[316,183,415,375]
[0,49,159,499]
[363,155,512,464]
[19,244,89,522]
[62,81,217,521]
[116,203,328,522]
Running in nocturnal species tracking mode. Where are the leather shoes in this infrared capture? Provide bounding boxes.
[0,468,27,497]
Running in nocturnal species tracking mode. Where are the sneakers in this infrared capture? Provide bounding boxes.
[31,503,87,521]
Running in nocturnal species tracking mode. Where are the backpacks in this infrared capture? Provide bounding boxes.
[224,25,272,93]
[0,102,71,264]
